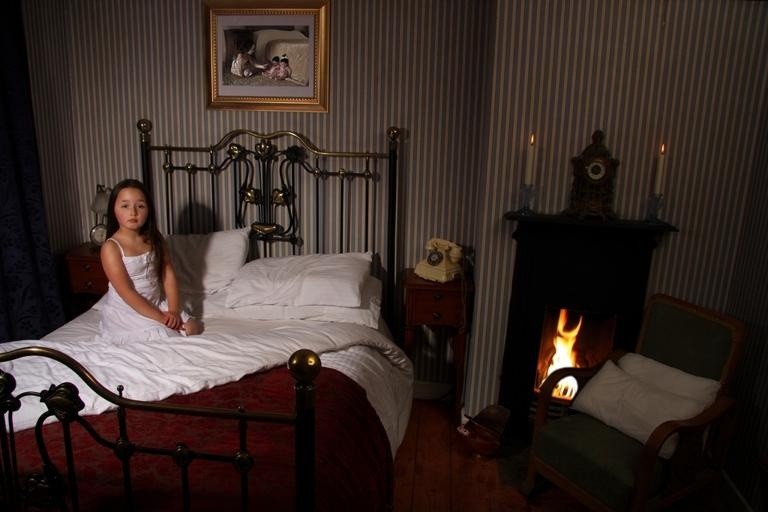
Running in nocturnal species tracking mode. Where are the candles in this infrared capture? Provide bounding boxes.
[652,143,665,195]
[522,133,535,186]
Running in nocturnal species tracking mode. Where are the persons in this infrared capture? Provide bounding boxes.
[95,179,182,341]
[231,42,292,80]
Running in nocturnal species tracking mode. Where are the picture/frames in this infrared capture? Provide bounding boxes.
[200,0,329,112]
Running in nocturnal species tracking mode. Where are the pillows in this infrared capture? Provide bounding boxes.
[158,225,384,331]
[616,352,722,455]
[569,359,707,461]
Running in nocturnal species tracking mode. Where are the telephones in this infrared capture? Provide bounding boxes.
[414,238,462,282]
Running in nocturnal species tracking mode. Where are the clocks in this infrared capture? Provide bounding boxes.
[89,224,107,248]
[559,128,622,221]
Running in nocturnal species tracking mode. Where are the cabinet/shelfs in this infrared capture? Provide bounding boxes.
[400,266,474,417]
[58,242,108,324]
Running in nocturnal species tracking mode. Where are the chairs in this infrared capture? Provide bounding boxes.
[521,294,745,512]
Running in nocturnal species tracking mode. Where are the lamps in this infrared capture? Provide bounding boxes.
[89,184,113,225]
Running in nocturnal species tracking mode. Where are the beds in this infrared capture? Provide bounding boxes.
[1,116,416,512]
